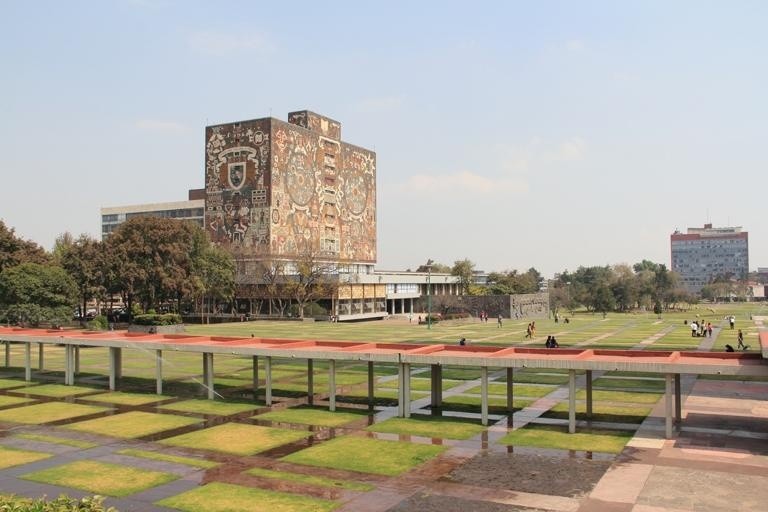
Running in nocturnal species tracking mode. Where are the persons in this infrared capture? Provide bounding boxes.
[460,338,465,345]
[526,321,537,339]
[497,314,503,328]
[690,315,745,352]
[565,318,569,323]
[554,311,559,322]
[545,335,559,348]
[480,311,489,323]
[420,315,421,322]
